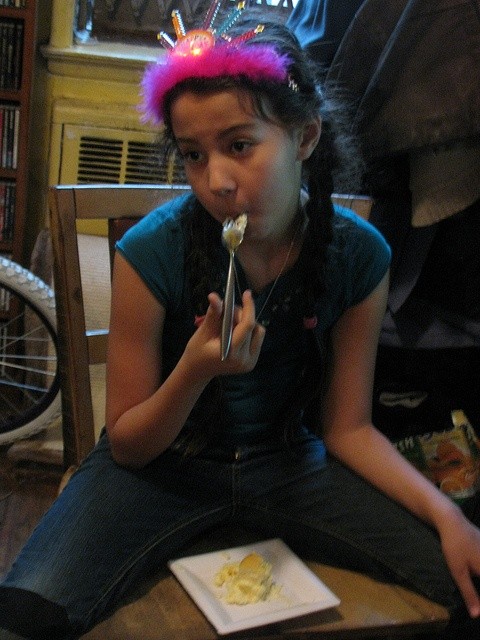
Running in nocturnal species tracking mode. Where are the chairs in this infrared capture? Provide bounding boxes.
[48,185,450,634]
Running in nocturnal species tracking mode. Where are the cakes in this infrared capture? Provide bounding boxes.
[219,551,280,604]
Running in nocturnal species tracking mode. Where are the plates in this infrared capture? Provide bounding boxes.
[167,538,340,634]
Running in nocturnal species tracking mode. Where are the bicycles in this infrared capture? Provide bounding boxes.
[0,258,61,445]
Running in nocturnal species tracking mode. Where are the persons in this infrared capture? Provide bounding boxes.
[1,9,480,640]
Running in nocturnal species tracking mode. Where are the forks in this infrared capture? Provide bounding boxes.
[220,214,247,359]
